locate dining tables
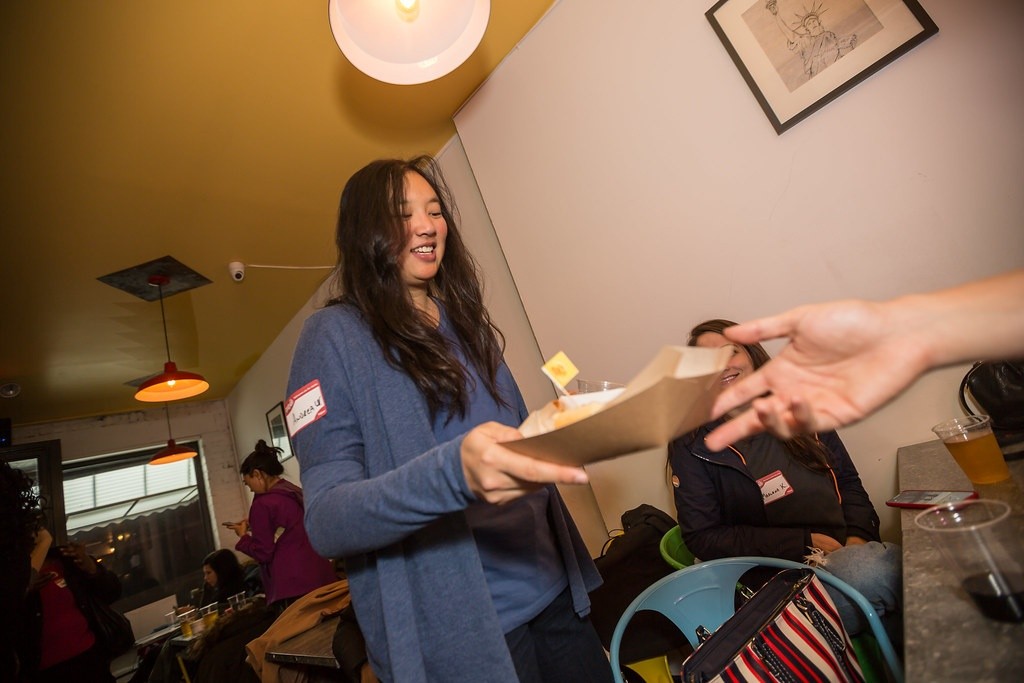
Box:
[134,613,355,683]
[896,439,1024,683]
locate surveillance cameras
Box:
[229,262,245,281]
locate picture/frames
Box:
[704,0,939,136]
[266,401,294,464]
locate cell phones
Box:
[886,488,979,511]
[222,521,238,526]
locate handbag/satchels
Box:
[959,357,1024,461]
[580,504,679,652]
[682,568,866,683]
[58,545,135,663]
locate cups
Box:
[165,610,178,629]
[176,601,219,637]
[576,378,626,393]
[227,590,247,614]
[931,414,1012,484]
[914,499,1024,623]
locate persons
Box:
[286,158,614,683]
[703,269,1024,453]
[200,549,253,614]
[0,462,121,683]
[667,319,902,630]
[226,439,330,619]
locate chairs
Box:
[127,594,180,640]
[609,525,903,683]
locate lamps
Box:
[329,0,490,86]
[134,272,209,402]
[148,402,198,464]
